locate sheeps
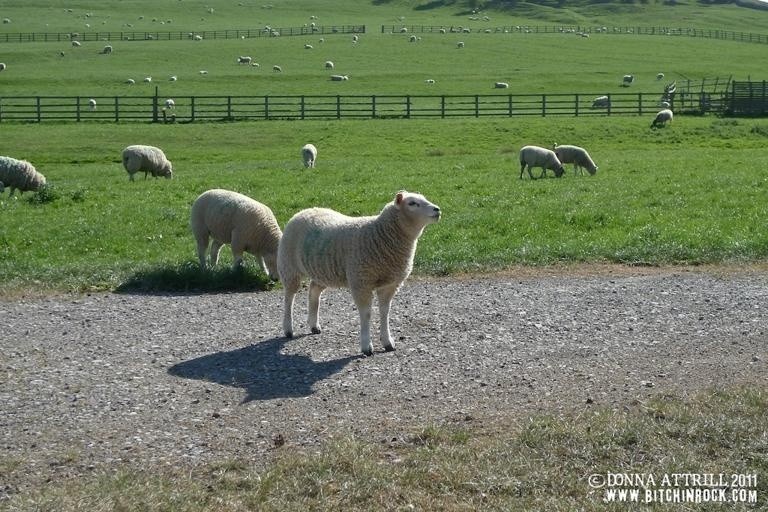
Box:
[518,142,598,180]
[650,109,674,128]
[592,95,608,108]
[301,144,317,169]
[121,144,174,182]
[191,188,284,283]
[276,189,442,356]
[494,80,508,90]
[0,156,46,197]
[657,73,664,79]
[1,25,466,110]
[622,74,634,83]
